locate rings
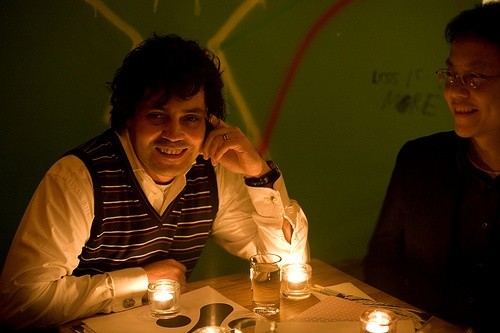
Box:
[222,133,228,139]
[214,120,226,131]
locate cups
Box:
[192,325,232,333]
[359,307,397,333]
[280,263,313,301]
[147,278,180,320]
[250,253,283,316]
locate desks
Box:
[58,259,475,333]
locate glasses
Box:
[436,68,500,89]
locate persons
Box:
[362,0,500,333]
[1,33,312,333]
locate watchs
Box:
[239,160,280,187]
